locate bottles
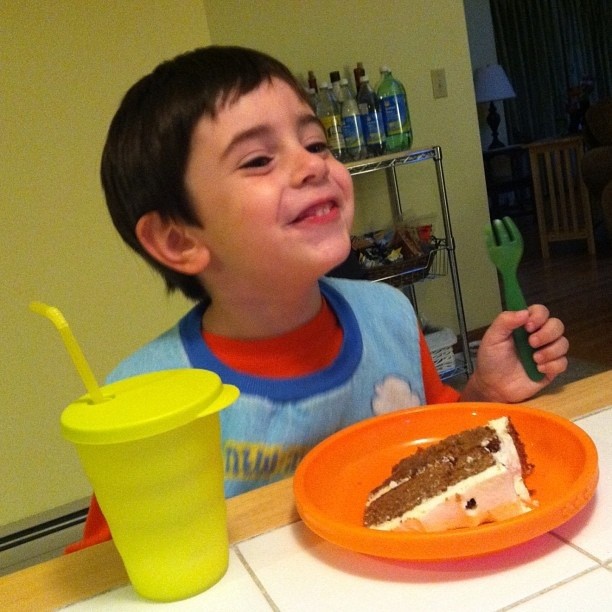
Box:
[356,75,386,158]
[307,88,318,114]
[337,79,369,161]
[305,70,320,103]
[329,71,344,113]
[354,69,378,102]
[372,66,391,93]
[317,82,346,162]
[327,83,341,124]
[377,69,413,151]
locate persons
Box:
[63,44,570,557]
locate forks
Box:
[485,217,543,382]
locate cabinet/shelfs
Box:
[343,144,473,388]
[483,143,536,220]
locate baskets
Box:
[424,325,458,376]
[344,235,437,288]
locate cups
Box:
[60,369,243,603]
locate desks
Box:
[520,132,612,262]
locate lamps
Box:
[475,61,519,147]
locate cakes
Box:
[361,414,539,533]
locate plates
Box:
[292,402,600,559]
